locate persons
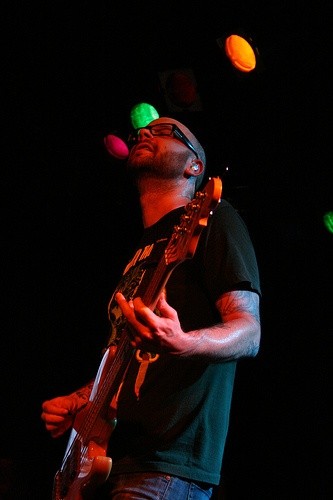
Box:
[39,110,264,499]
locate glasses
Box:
[127,123,200,161]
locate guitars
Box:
[53,177,223,500]
